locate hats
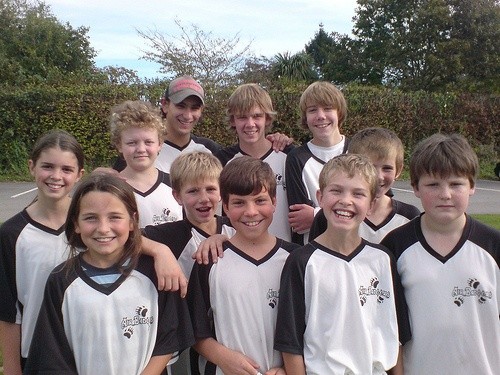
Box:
[165,74,205,107]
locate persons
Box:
[104,102,187,228]
[0,129,500,375]
[286,79,362,247]
[110,75,296,174]
[214,85,296,244]
